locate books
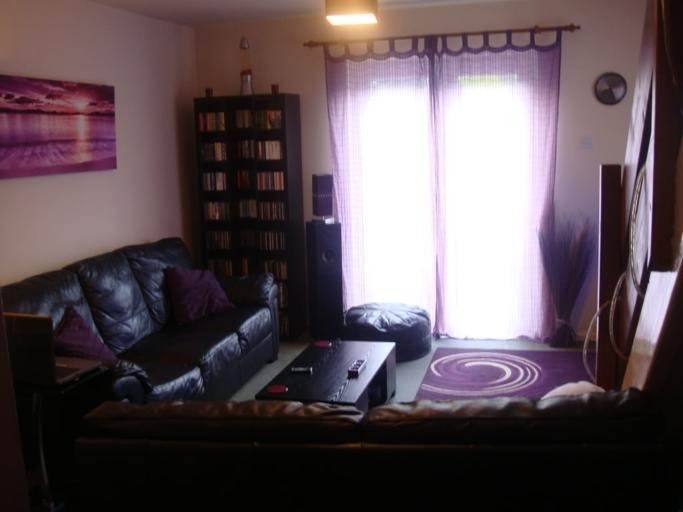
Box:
[198,109,298,338]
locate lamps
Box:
[324,1,379,27]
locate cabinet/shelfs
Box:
[193,92,307,339]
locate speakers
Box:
[306,222,344,336]
[312,174,333,216]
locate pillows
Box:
[51,304,153,394]
[164,264,236,327]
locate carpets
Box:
[412,346,596,402]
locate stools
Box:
[345,303,431,362]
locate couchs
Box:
[69,378,680,512]
[0,237,279,509]
